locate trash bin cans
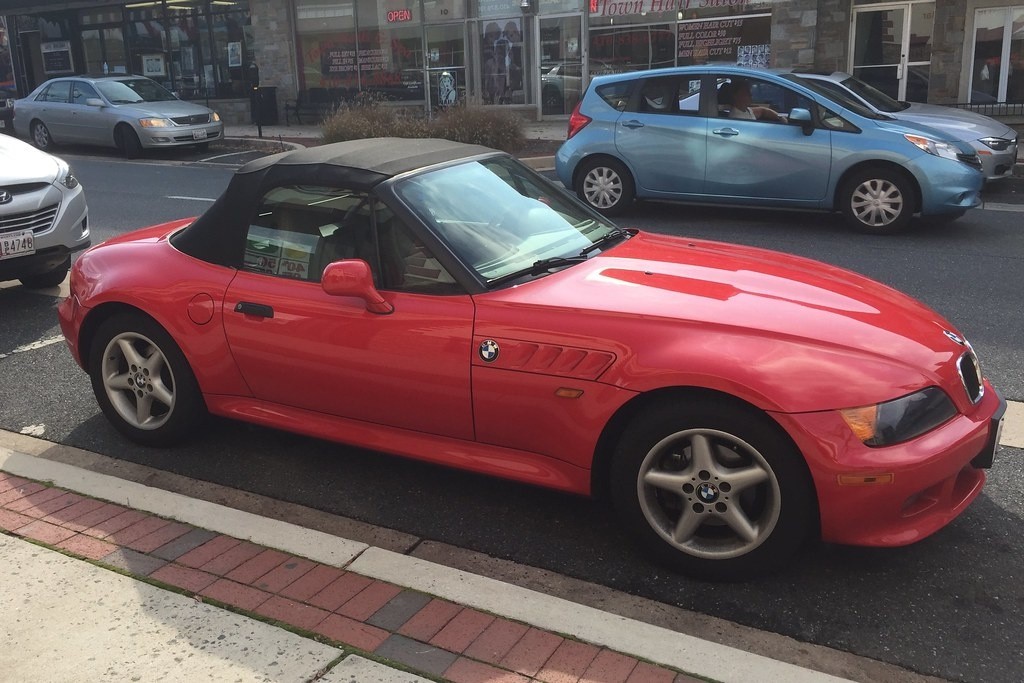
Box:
[250,86,278,126]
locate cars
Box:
[553,62,982,237]
[0,129,90,289]
[854,60,998,107]
[57,136,1008,582]
[0,89,16,131]
[9,72,227,158]
[660,69,1021,189]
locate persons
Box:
[727,75,790,126]
[480,20,527,105]
